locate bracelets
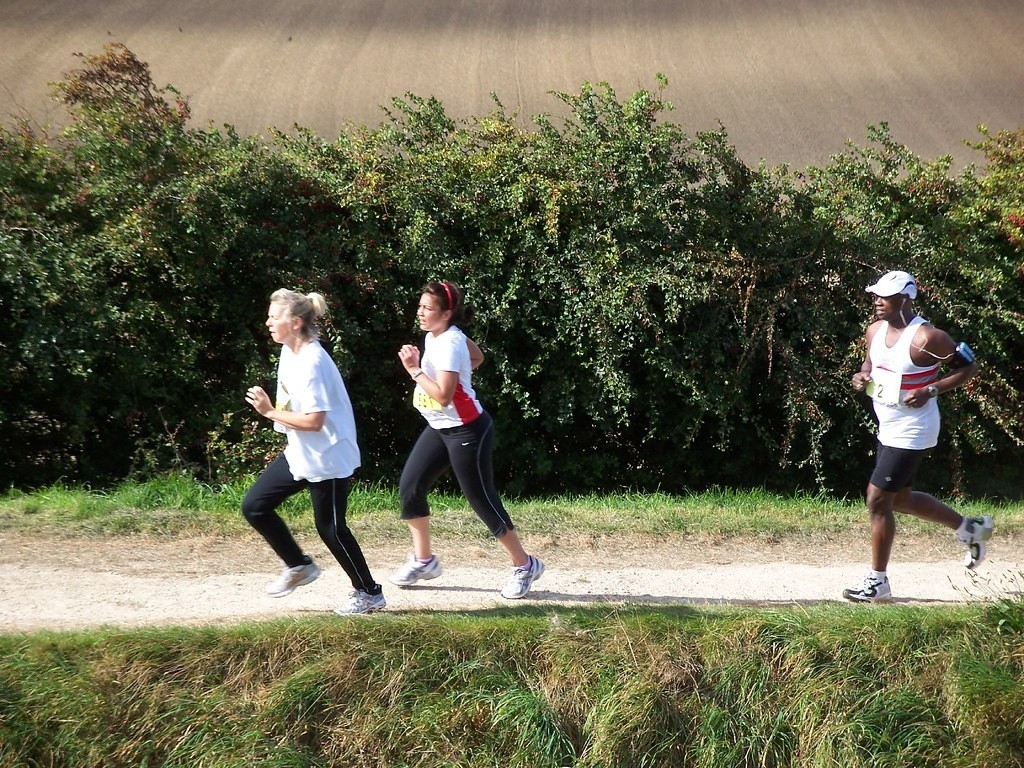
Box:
[411,369,423,379]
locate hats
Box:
[865,270,918,300]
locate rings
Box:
[856,384,860,388]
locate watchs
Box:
[927,385,938,398]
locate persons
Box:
[842,271,992,603]
[389,282,545,599]
[240,288,387,617]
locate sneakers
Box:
[964,515,992,570]
[333,590,387,616]
[843,575,891,603]
[390,553,443,586]
[265,562,321,599]
[500,555,546,599]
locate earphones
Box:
[902,298,907,302]
